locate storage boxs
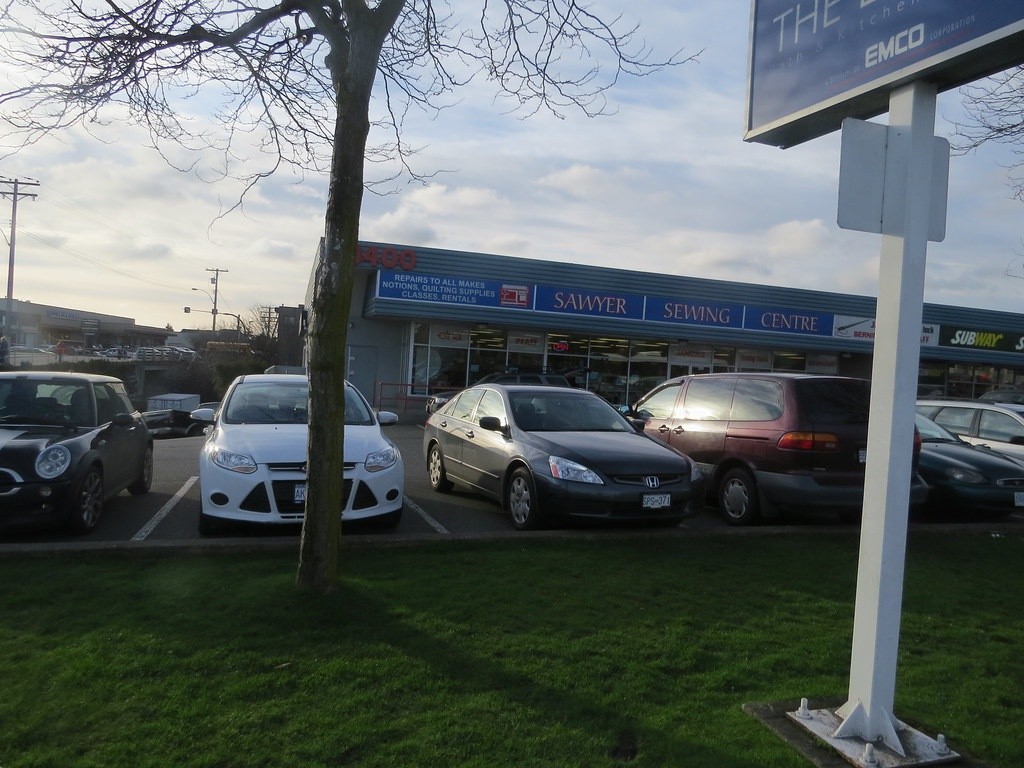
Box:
[147,393,200,412]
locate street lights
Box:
[192,288,218,332]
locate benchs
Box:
[269,400,307,420]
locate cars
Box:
[422,382,708,533]
[910,409,1024,521]
[187,372,405,537]
[0,370,155,535]
[10,340,196,362]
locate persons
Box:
[117,347,128,360]
[68,388,99,426]
[0,380,49,423]
[57,339,64,363]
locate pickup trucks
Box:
[139,401,221,440]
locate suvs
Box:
[610,372,922,527]
[979,388,1024,406]
[424,366,578,418]
[916,395,1024,461]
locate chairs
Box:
[66,389,107,427]
[237,393,275,421]
[516,403,543,431]
[0,385,46,419]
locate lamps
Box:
[348,321,353,328]
